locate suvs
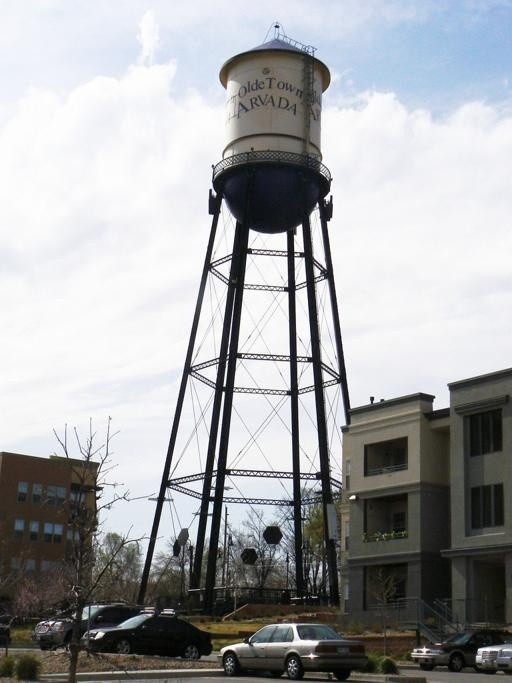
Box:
[34,602,142,650]
[476,634,512,675]
[410,630,512,673]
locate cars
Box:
[81,613,213,660]
[216,621,369,680]
